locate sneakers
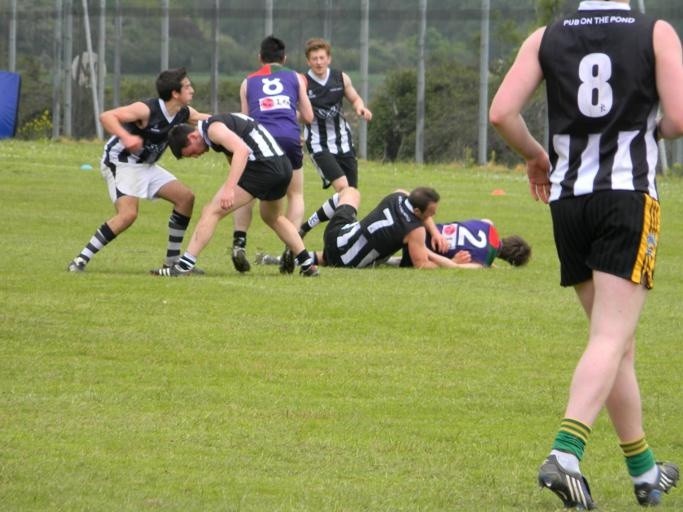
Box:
[538,454,593,511]
[151,261,204,276]
[634,461,679,507]
[68,261,83,273]
[231,246,319,276]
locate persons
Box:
[66,66,212,276]
[384,217,531,270]
[299,38,372,193]
[254,185,471,270]
[149,111,320,277]
[490,0,683,512]
[240,35,315,275]
